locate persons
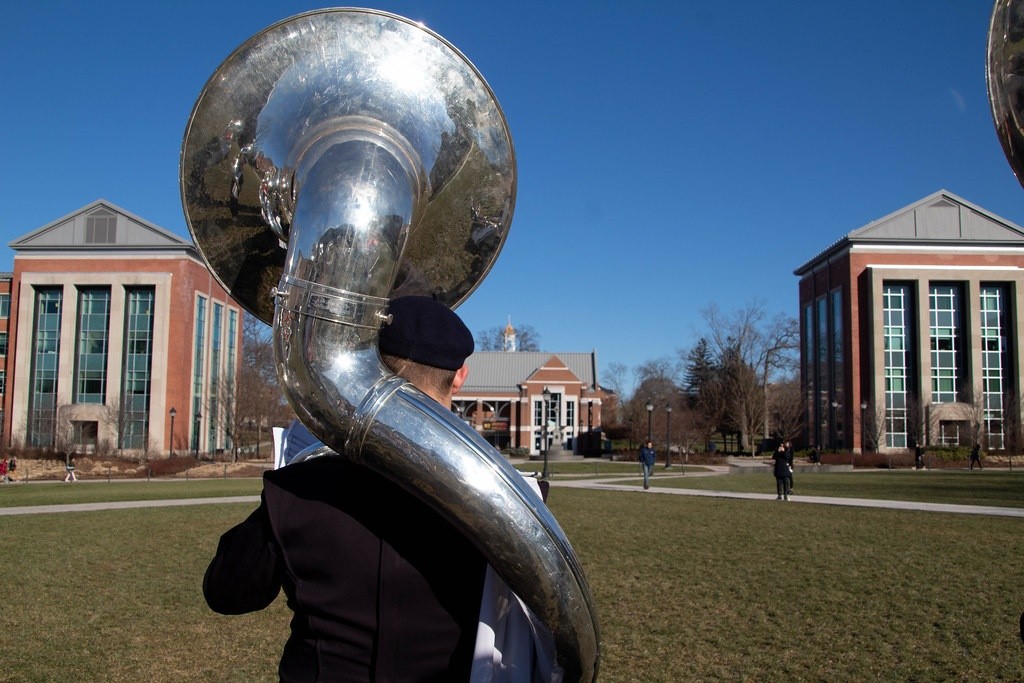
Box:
[65,457,78,482]
[0,458,8,482]
[784,440,794,494]
[202,295,558,683]
[970,444,982,470]
[9,456,19,481]
[810,447,821,466]
[640,441,656,489]
[772,438,791,500]
[912,441,926,470]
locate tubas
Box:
[176,5,603,682]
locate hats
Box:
[378,293,474,371]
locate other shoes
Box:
[644,485,648,489]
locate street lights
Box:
[665,402,673,469]
[195,412,202,461]
[170,407,176,458]
[542,388,552,480]
[645,398,654,442]
[861,401,867,454]
[832,398,838,452]
